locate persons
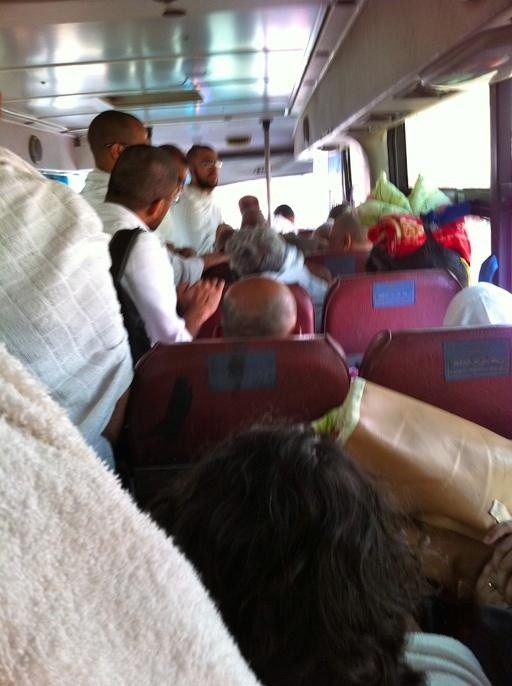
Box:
[2,146,134,475]
[163,421,489,683]
[213,277,303,338]
[2,341,262,683]
[78,111,151,207]
[225,224,332,334]
[153,145,234,258]
[238,196,374,257]
[359,170,471,288]
[482,520,510,606]
[444,281,510,328]
[96,145,230,367]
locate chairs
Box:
[359,323,511,435]
[124,336,351,464]
[325,269,464,357]
[117,228,377,338]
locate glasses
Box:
[200,160,225,170]
